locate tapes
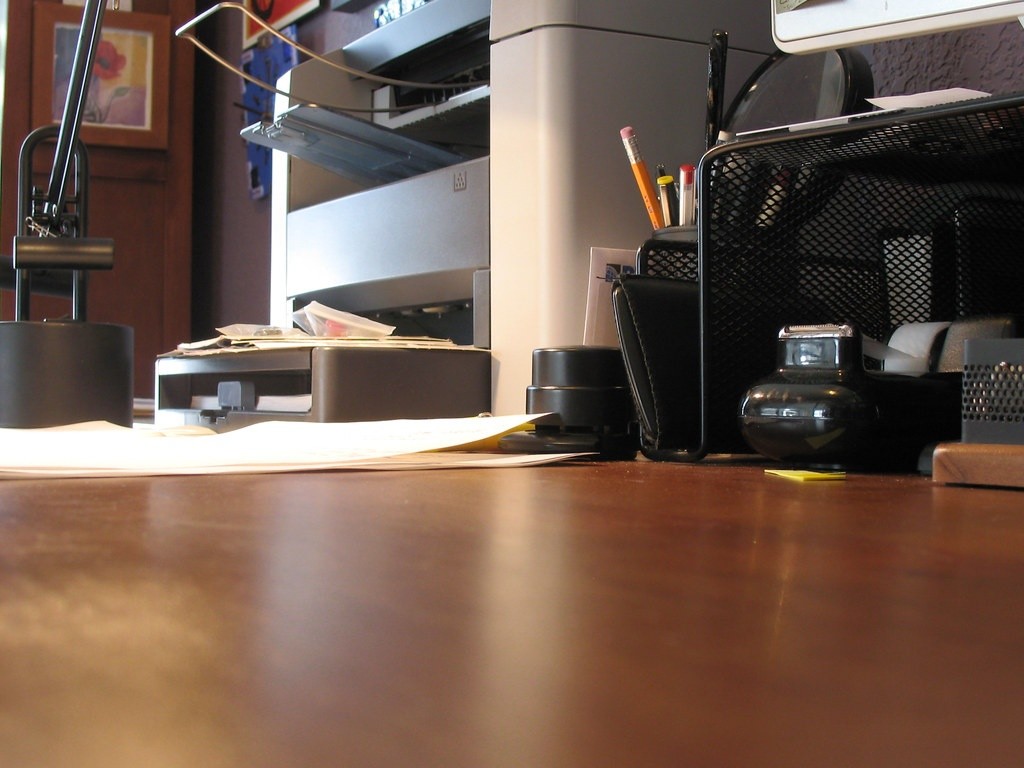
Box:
[882,321,952,376]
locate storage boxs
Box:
[962,337,1024,445]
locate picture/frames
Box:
[241,0,324,51]
[30,2,172,151]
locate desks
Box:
[0,409,1024,768]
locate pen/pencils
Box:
[620,29,736,231]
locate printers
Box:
[154,0,826,440]
